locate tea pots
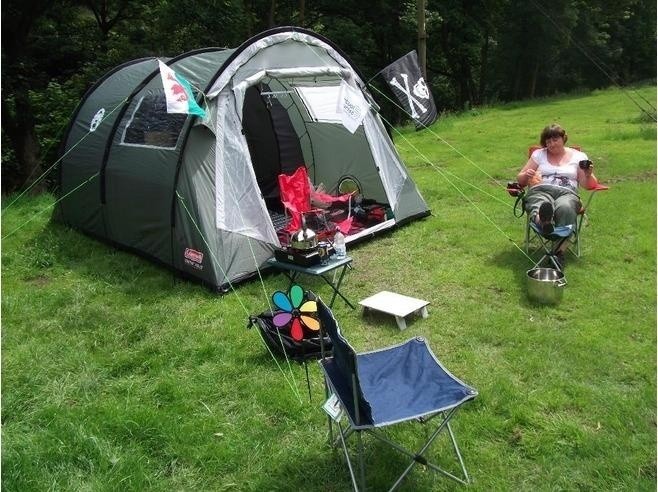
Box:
[290,215,318,252]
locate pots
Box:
[526,267,567,304]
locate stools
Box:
[358,289,432,331]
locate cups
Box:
[579,160,590,169]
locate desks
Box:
[267,254,355,313]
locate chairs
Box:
[507,145,609,278]
[313,295,482,490]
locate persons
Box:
[517,125,598,258]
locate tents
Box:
[51,26,431,293]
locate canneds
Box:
[318,243,328,266]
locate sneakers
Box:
[539,200,555,234]
[551,250,566,269]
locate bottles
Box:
[332,225,347,260]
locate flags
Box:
[335,79,372,135]
[381,50,440,131]
[158,59,207,118]
